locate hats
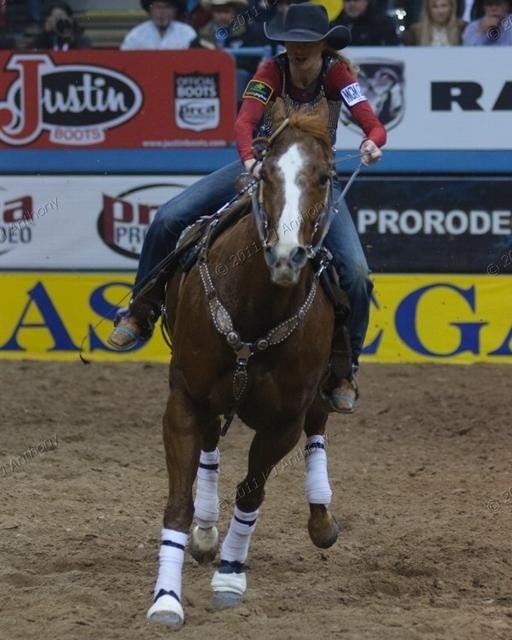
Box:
[200,0,248,13]
[262,4,351,51]
[140,0,187,16]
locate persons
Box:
[119,1,511,50]
[106,1,389,416]
[0,1,90,49]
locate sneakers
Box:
[328,377,359,415]
[107,311,152,351]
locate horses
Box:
[145,96,339,630]
